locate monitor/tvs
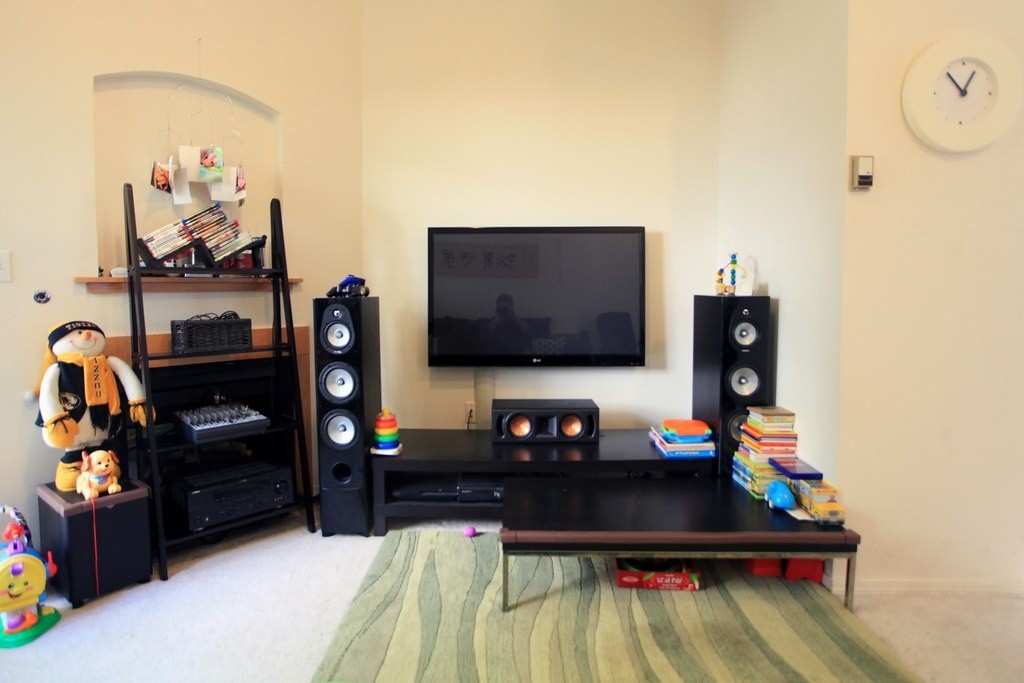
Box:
[427,228,646,367]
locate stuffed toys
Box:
[25,321,156,502]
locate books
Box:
[142,204,252,262]
[732,406,797,500]
[648,426,716,459]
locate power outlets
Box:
[463,401,477,423]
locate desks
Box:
[498,476,862,614]
[370,428,716,535]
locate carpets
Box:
[310,529,926,683]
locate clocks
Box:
[900,30,1024,155]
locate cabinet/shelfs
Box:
[122,183,317,582]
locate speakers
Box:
[491,399,600,445]
[692,295,773,477]
[313,296,382,533]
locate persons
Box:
[235,175,246,193]
[200,148,223,179]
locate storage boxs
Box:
[614,558,701,591]
